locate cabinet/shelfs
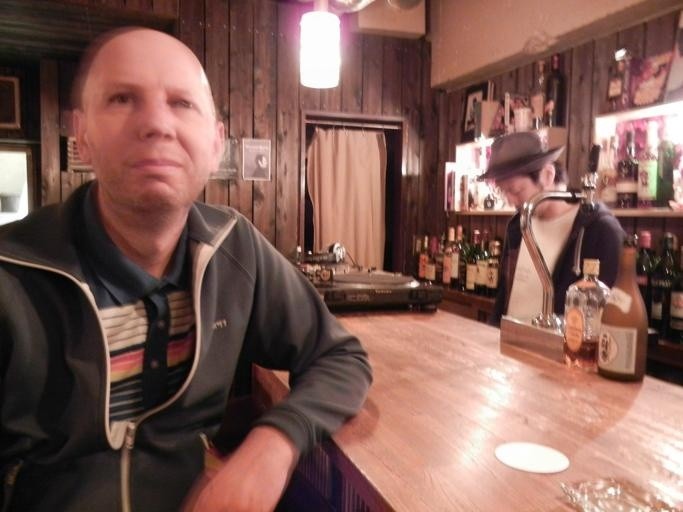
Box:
[448,125,567,217]
[435,286,682,387]
[585,96,681,219]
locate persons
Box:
[476,131,628,328]
[252,155,267,178]
[0,26,375,512]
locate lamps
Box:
[297,0,341,90]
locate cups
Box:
[512,107,532,132]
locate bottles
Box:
[594,234,647,384]
[562,259,610,369]
[580,130,682,208]
[636,230,682,347]
[531,53,567,127]
[413,224,501,296]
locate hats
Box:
[477,132,566,181]
